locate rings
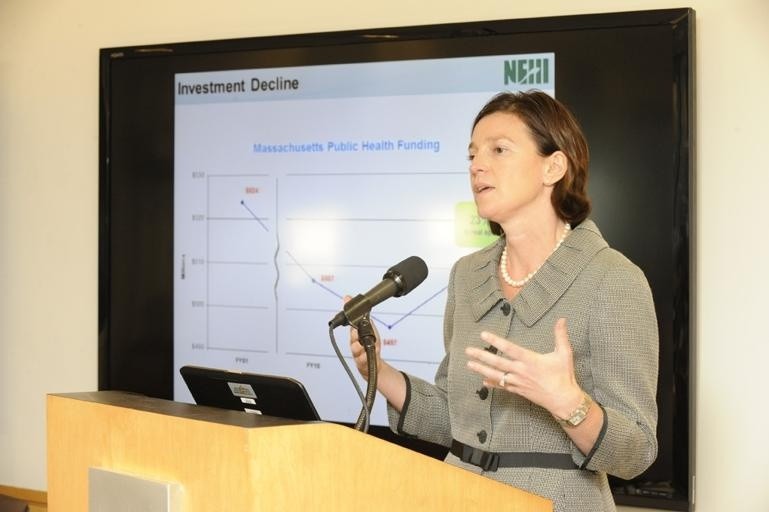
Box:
[498,369,513,390]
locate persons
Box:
[343,87,659,512]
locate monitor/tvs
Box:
[98,6,695,512]
[179,365,321,421]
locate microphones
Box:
[328,256,429,330]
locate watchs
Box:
[552,391,594,430]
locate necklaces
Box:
[499,219,573,288]
